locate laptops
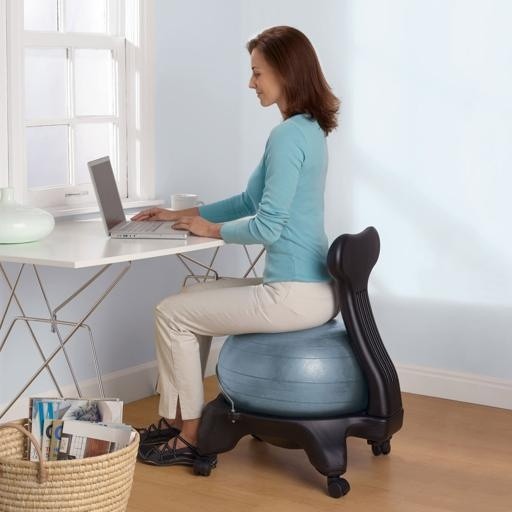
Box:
[87,155,191,240]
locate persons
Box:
[130,26,342,468]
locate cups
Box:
[171,194,204,210]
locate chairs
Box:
[192,227,405,500]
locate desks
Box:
[1,208,266,423]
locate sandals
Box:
[132,415,182,444]
[138,433,218,468]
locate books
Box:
[26,398,134,465]
